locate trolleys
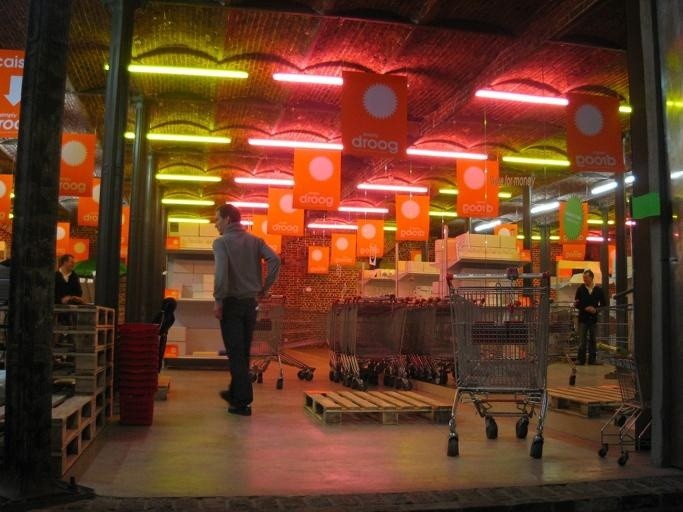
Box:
[326,295,488,392]
[248,294,316,390]
[592,302,652,466]
[517,300,579,386]
[445,266,551,459]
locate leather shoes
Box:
[574,359,585,365]
[220,390,239,406]
[227,406,253,416]
[588,360,600,365]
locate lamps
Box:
[586,169,638,200]
[404,145,490,162]
[127,62,252,81]
[148,166,516,241]
[122,128,234,147]
[518,191,565,219]
[472,85,570,107]
[501,153,571,170]
[269,67,412,92]
[616,104,635,116]
[245,132,343,153]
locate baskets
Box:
[115,322,160,427]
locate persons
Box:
[572,269,608,366]
[210,202,281,417]
[54,253,88,365]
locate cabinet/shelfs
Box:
[51,306,115,477]
